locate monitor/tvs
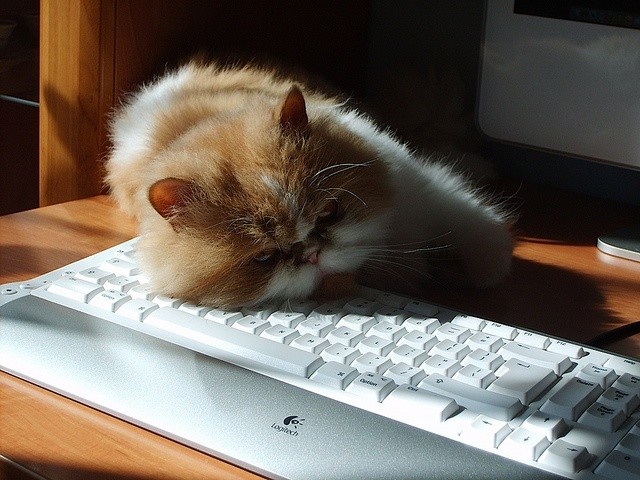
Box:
[474,1,639,261]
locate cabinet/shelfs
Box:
[1,1,210,210]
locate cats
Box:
[102,52,526,312]
